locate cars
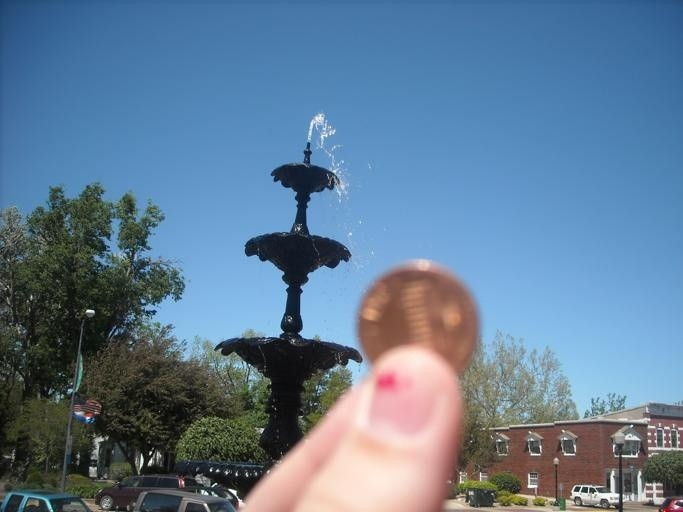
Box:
[657,496,683,512]
[570,485,626,509]
[0,475,239,512]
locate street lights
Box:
[60,308,95,493]
[614,430,625,512]
[553,456,560,505]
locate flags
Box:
[73,398,101,425]
[75,353,82,392]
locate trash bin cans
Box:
[468,488,496,507]
[560,497,566,510]
[89,460,98,480]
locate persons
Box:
[236,345,464,511]
